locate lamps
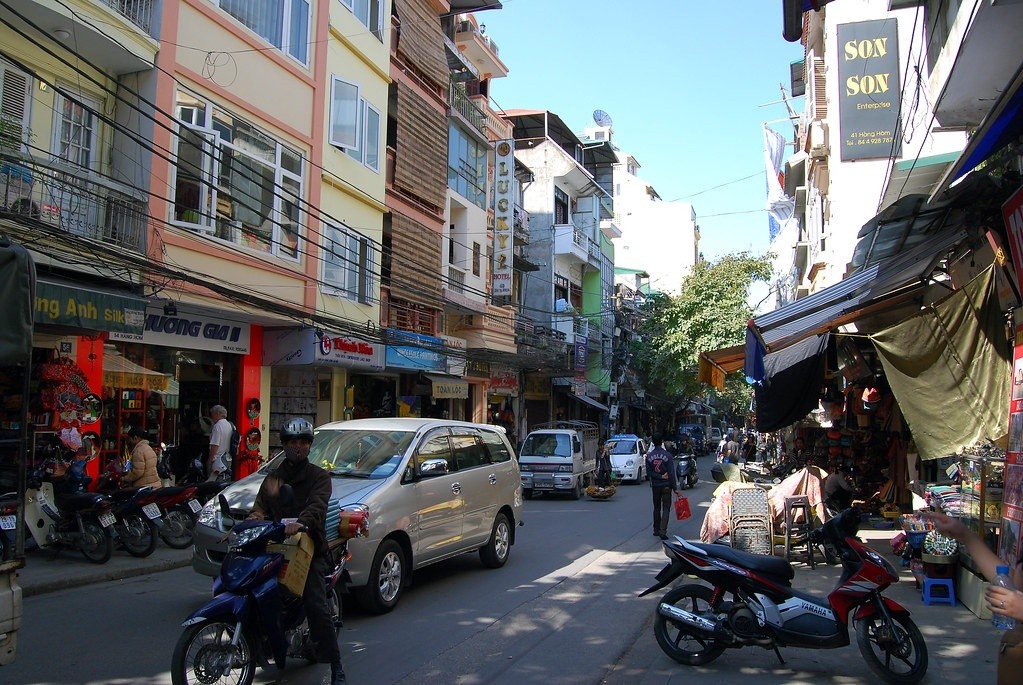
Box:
[163,298,178,315]
[479,22,486,35]
[51,27,73,41]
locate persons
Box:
[824,465,854,508]
[789,437,816,471]
[119,427,162,492]
[922,511,1023,685]
[221,418,347,685]
[675,438,696,478]
[646,432,682,540]
[715,433,769,465]
[596,437,612,500]
[207,405,242,482]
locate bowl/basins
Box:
[921,552,957,578]
[905,530,928,547]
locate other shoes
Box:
[659,533,669,540]
[653,532,659,536]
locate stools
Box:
[783,495,817,570]
[921,576,958,608]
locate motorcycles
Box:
[170,492,352,685]
[0,441,236,565]
[638,491,929,685]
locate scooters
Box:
[673,453,700,490]
[710,452,862,565]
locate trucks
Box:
[675,414,713,457]
[517,419,600,500]
[710,419,726,452]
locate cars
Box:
[606,439,649,485]
[191,417,525,614]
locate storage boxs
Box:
[266,531,315,598]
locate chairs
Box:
[728,487,775,556]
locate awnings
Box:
[34,275,149,335]
[423,375,469,399]
[699,222,968,392]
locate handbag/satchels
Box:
[231,423,238,454]
[674,493,691,520]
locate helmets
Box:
[279,418,315,439]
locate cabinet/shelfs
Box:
[960,453,1005,575]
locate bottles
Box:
[992,565,1016,631]
